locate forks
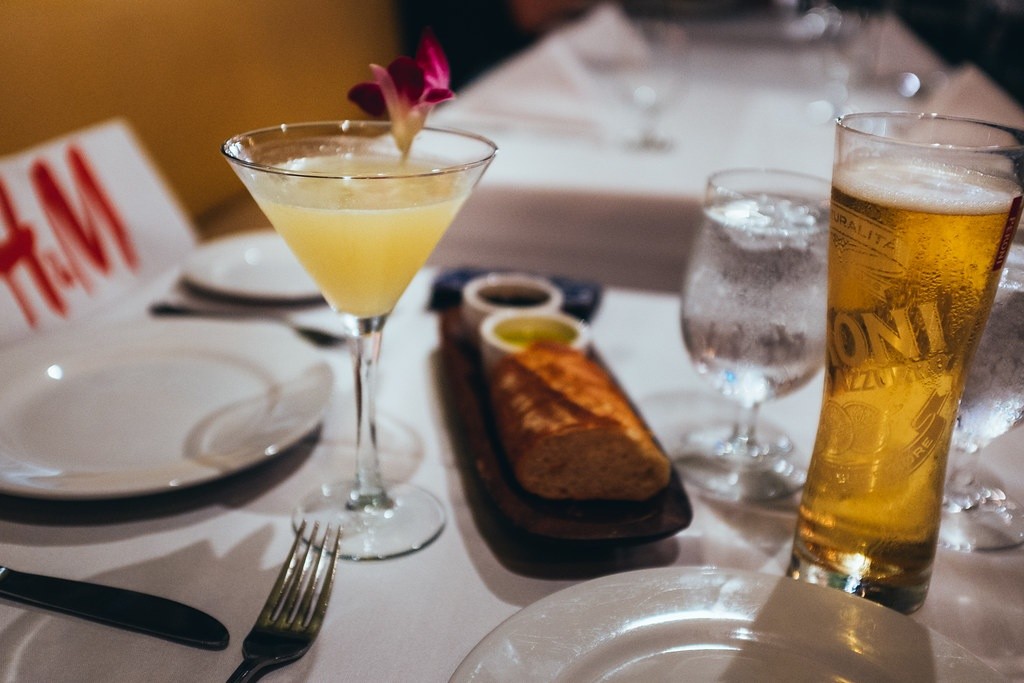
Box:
[225,519,343,683]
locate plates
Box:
[448,566,1014,683]
[182,230,322,301]
[443,289,693,544]
[0,317,336,498]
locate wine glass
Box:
[928,237,1024,552]
[219,121,500,560]
[679,169,838,501]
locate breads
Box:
[485,338,671,502]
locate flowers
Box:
[345,37,457,158]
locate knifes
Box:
[1,566,229,652]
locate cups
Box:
[786,112,1024,616]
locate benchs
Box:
[0,0,398,242]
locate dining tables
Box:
[0,5,1024,683]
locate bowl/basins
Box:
[480,311,592,368]
[463,271,564,333]
